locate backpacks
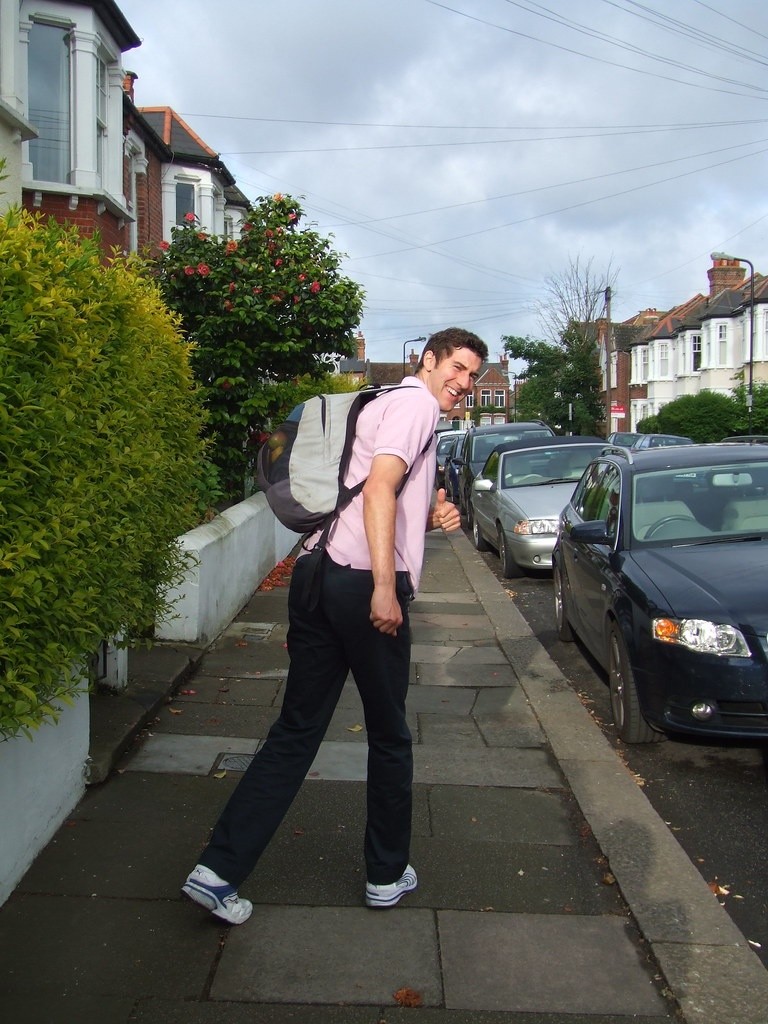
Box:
[256,384,433,532]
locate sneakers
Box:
[366,864,417,908]
[181,864,253,925]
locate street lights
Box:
[402,336,427,379]
[710,251,754,435]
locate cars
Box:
[435,429,465,506]
[469,436,616,579]
[717,436,768,447]
[606,431,645,453]
[629,434,705,494]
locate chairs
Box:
[634,500,696,539]
[563,451,592,478]
[721,495,768,532]
[510,458,532,474]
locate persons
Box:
[178,328,488,925]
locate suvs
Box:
[548,435,768,745]
[450,420,556,533]
